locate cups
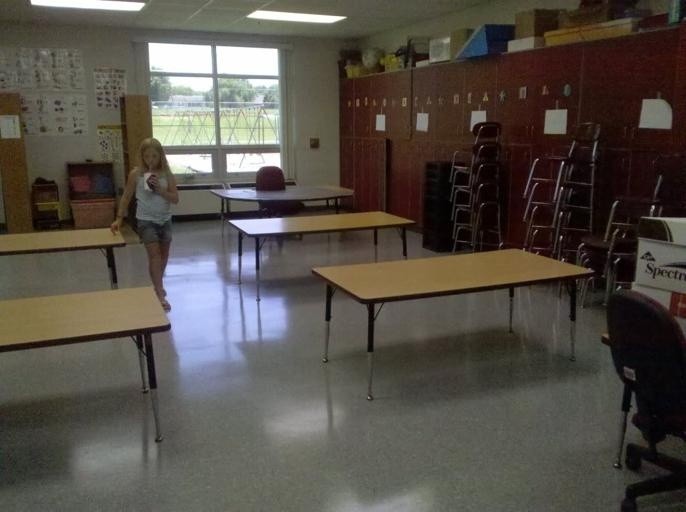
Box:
[144,173,158,191]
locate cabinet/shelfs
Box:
[1,92,33,235]
[339,69,413,225]
[118,94,153,231]
[414,25,686,286]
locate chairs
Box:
[602,286,686,511]
[257,166,302,240]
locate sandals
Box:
[159,294,171,311]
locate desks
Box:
[310,248,593,400]
[71,200,116,231]
[211,182,357,250]
[0,285,170,446]
[227,212,417,302]
[0,226,128,287]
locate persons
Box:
[109,137,179,312]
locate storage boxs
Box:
[427,2,670,62]
[30,183,59,232]
[631,214,686,338]
